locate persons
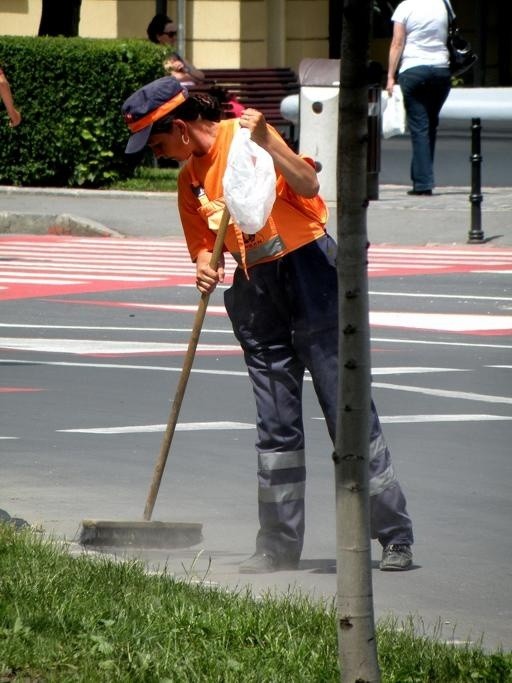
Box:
[0,68,22,128]
[384,0,456,196]
[122,74,415,577]
[146,12,207,169]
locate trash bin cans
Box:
[299,56,385,202]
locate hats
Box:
[124,77,189,155]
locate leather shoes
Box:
[407,188,432,196]
[379,544,415,570]
[238,553,300,575]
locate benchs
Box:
[152,67,302,169]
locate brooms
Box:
[81,204,229,551]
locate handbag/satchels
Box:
[447,17,480,78]
[379,84,407,139]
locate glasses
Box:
[162,30,178,39]
